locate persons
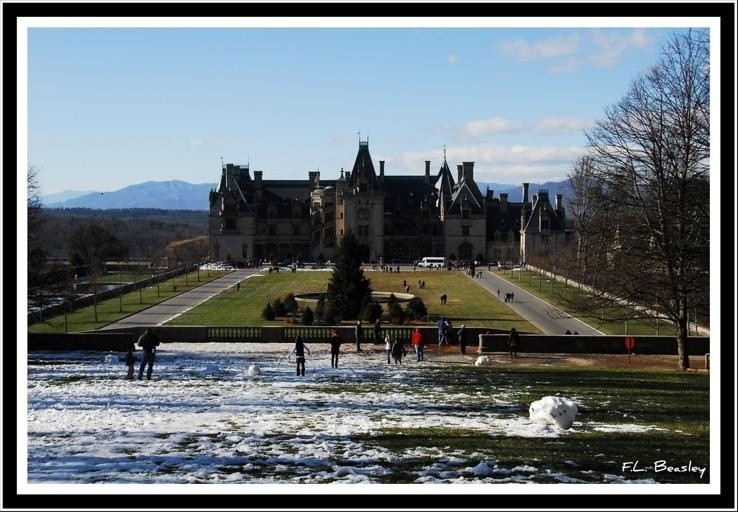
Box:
[355,315,468,365]
[565,329,571,335]
[403,280,425,293]
[124,346,137,378]
[329,331,341,368]
[136,331,159,380]
[441,295,447,304]
[413,252,517,279]
[497,288,514,303]
[506,327,520,358]
[574,331,579,335]
[370,263,400,274]
[288,335,310,376]
[241,256,301,274]
[237,283,240,291]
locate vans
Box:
[498,262,511,269]
[418,256,446,268]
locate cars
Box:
[512,265,527,272]
[218,264,234,270]
[488,260,497,266]
